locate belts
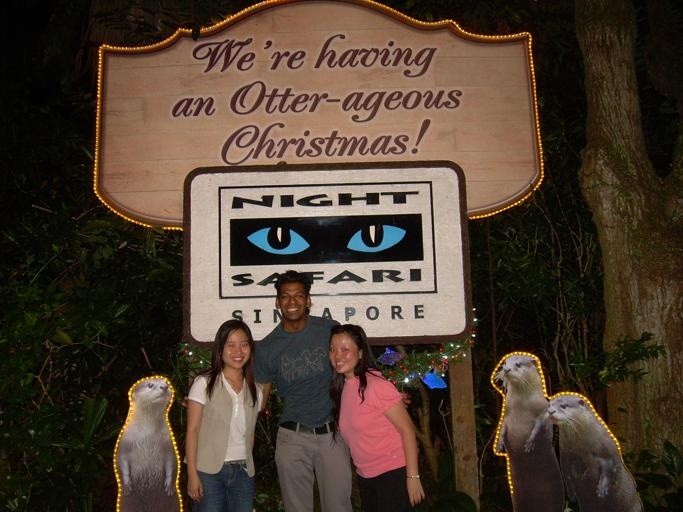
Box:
[279,420,336,435]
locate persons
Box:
[327,323,427,512]
[180,269,412,510]
[179,319,264,512]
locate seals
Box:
[116,377,180,512]
[545,393,644,511]
[492,354,568,512]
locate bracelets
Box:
[405,475,419,479]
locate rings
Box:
[192,494,196,497]
